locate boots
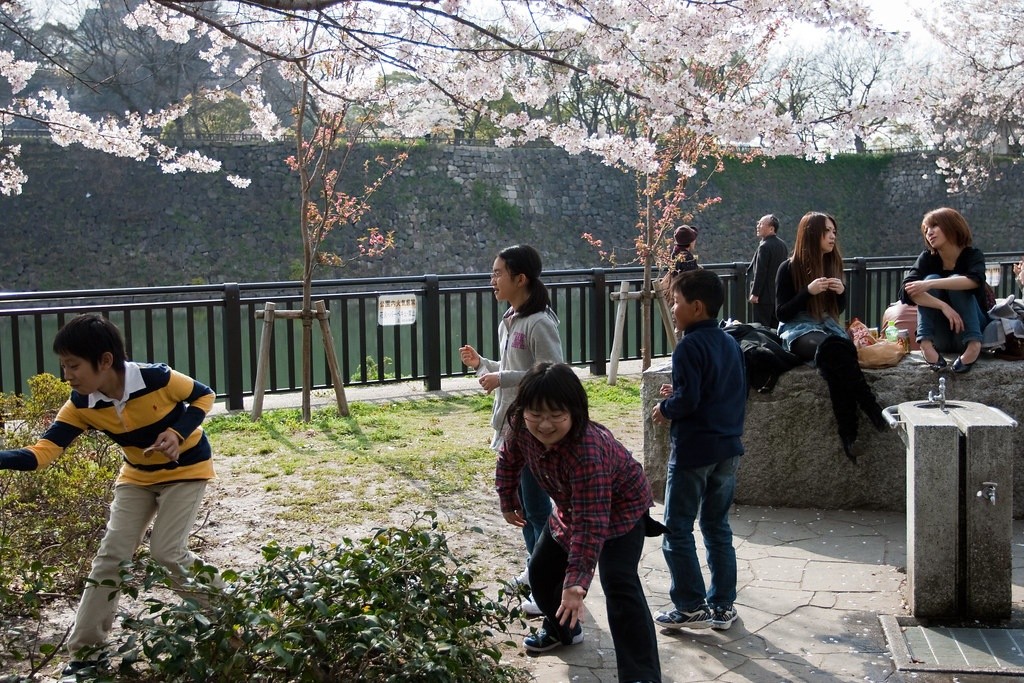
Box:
[814,335,891,458]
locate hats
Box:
[673,225,700,245]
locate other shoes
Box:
[921,346,948,373]
[518,593,545,615]
[950,354,979,374]
[503,567,531,594]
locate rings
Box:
[835,284,839,288]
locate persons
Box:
[900,206,990,373]
[647,269,751,634]
[774,208,896,462]
[494,358,676,683]
[748,212,787,334]
[1011,253,1024,301]
[663,224,708,341]
[453,243,584,656]
[2,312,241,683]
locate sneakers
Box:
[711,606,737,630]
[653,604,713,630]
[522,621,583,651]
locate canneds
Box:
[897,329,912,354]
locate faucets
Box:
[928,376,949,413]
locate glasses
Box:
[522,409,571,424]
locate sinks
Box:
[912,399,970,415]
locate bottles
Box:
[885,321,898,344]
